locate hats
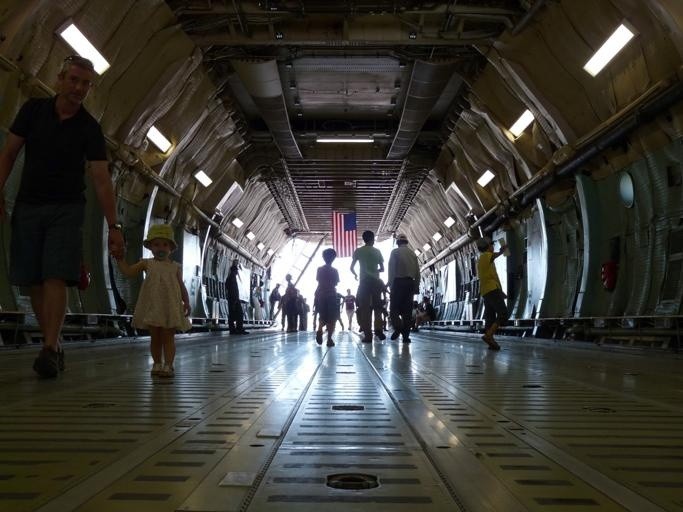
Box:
[396,234,409,243]
[476,237,497,247]
[142,224,179,251]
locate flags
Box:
[331,210,358,258]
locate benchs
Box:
[1,285,138,350]
[473,292,683,357]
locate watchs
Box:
[107,221,123,230]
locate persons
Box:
[108,223,193,379]
[384,233,421,344]
[224,265,250,336]
[313,247,340,346]
[475,236,510,351]
[349,231,386,343]
[0,52,129,381]
[249,274,436,333]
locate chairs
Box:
[418,300,470,331]
[190,296,275,331]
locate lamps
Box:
[582,18,639,79]
[54,18,112,78]
[192,168,212,188]
[244,230,255,241]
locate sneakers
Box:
[162,366,174,377]
[403,337,412,344]
[230,329,249,335]
[33,352,61,379]
[481,335,500,350]
[361,335,372,343]
[327,341,335,347]
[390,331,399,340]
[286,329,306,333]
[376,334,386,341]
[151,363,161,373]
[316,331,322,344]
